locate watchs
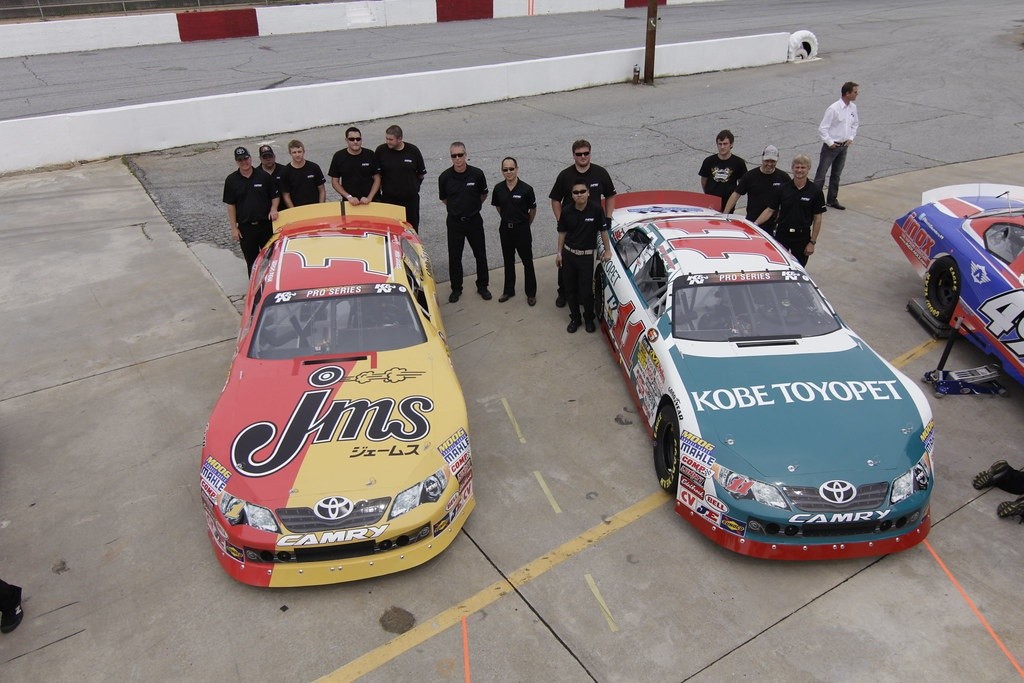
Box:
[809,241,816,245]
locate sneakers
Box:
[973,460,1009,490]
[997,496,1024,518]
[556,296,567,308]
[448,291,462,303]
[477,288,492,300]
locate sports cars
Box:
[200,201,478,588]
[594,190,934,561]
[891,182,1024,392]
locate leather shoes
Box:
[499,294,515,302]
[528,297,536,306]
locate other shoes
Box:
[567,313,582,333]
[583,311,597,333]
[826,196,846,210]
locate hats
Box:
[234,146,248,160]
[259,145,274,157]
[762,145,779,161]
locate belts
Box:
[238,219,269,225]
[564,244,593,256]
[500,221,527,228]
[834,142,846,147]
[778,224,810,233]
[448,214,480,221]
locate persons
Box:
[549,140,616,307]
[438,142,493,303]
[255,144,286,212]
[555,181,611,333]
[753,156,826,268]
[327,127,382,206]
[699,130,748,214]
[222,147,281,279]
[0,579,23,633]
[813,82,859,210]
[492,157,537,307]
[723,145,791,236]
[375,125,427,235]
[280,139,326,209]
[973,460,1024,525]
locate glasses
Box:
[261,154,274,159]
[572,189,588,194]
[237,156,249,161]
[451,153,465,158]
[575,151,591,156]
[347,137,362,142]
[503,167,516,172]
